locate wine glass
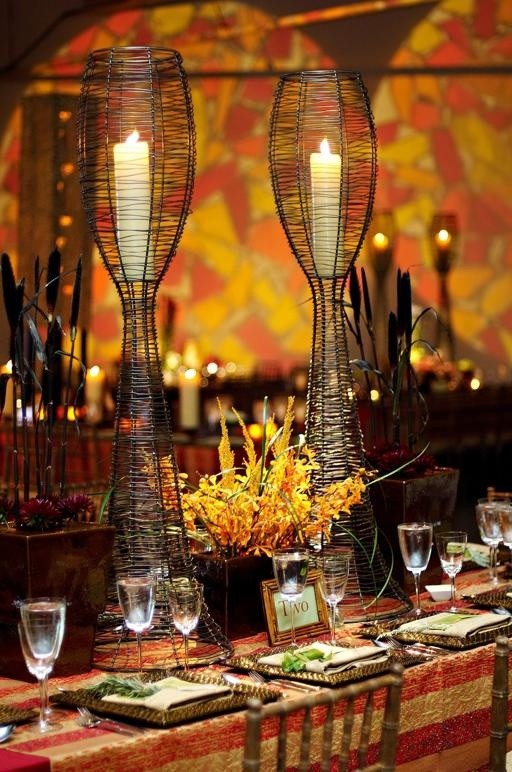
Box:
[434,531,468,614]
[270,547,310,646]
[168,581,205,673]
[17,595,69,733]
[475,496,512,588]
[117,577,158,674]
[316,555,351,645]
[397,522,435,616]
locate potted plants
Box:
[1,248,114,678]
[324,258,460,600]
[137,397,376,646]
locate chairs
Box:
[242,664,408,772]
[487,488,511,508]
[487,633,512,772]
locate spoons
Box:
[370,639,430,656]
[0,724,17,742]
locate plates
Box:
[423,583,458,602]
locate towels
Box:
[101,675,233,715]
[257,639,389,675]
[396,611,511,637]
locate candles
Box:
[113,129,155,281]
[306,137,345,276]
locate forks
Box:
[247,670,317,696]
[385,634,450,656]
[78,705,150,737]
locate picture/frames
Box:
[260,569,331,647]
